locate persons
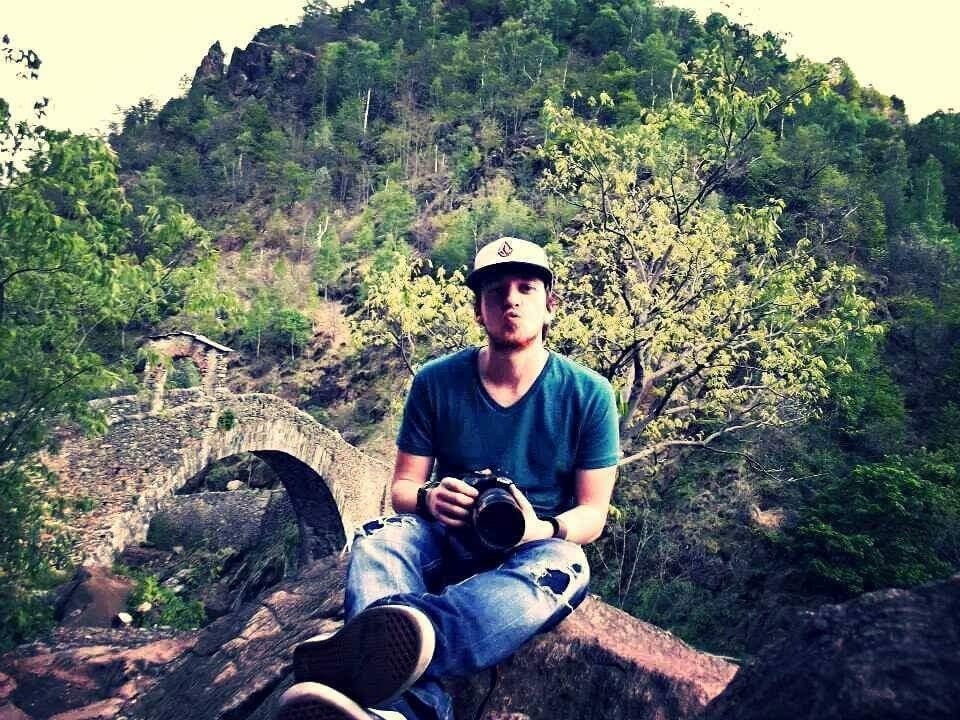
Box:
[278,237,620,720]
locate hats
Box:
[464,237,557,285]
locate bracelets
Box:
[540,516,560,537]
[415,480,440,523]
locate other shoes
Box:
[272,682,407,720]
[291,603,435,708]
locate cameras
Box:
[460,469,526,553]
